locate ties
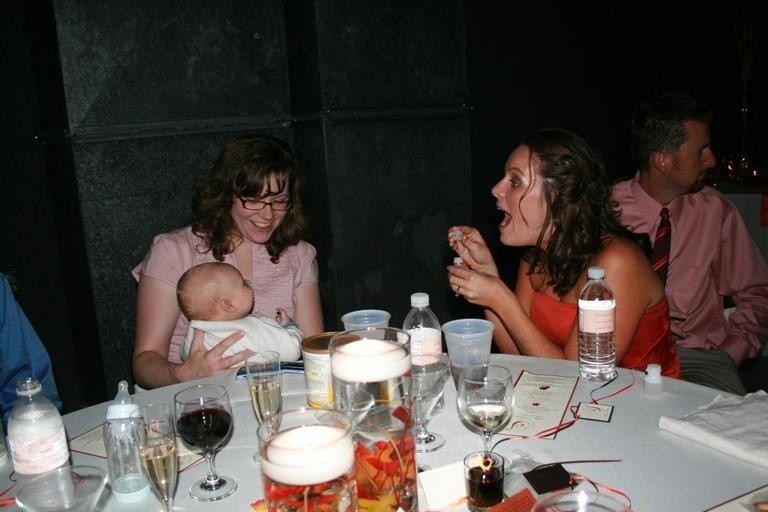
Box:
[650,208,671,288]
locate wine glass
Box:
[131,399,184,512]
[244,348,282,463]
[456,364,516,449]
[171,380,239,502]
[398,349,445,452]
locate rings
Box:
[455,286,460,297]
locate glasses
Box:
[236,195,293,211]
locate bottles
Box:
[576,267,617,381]
[453,257,469,297]
[642,363,665,397]
[101,380,151,503]
[7,376,76,508]
[400,291,442,371]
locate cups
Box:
[463,450,504,512]
[327,326,419,512]
[256,407,358,511]
[16,462,107,511]
[529,488,629,511]
[439,318,496,390]
[338,308,393,339]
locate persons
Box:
[446,126,680,378]
[176,263,305,374]
[606,90,768,396]
[0,270,63,430]
[129,134,326,389]
